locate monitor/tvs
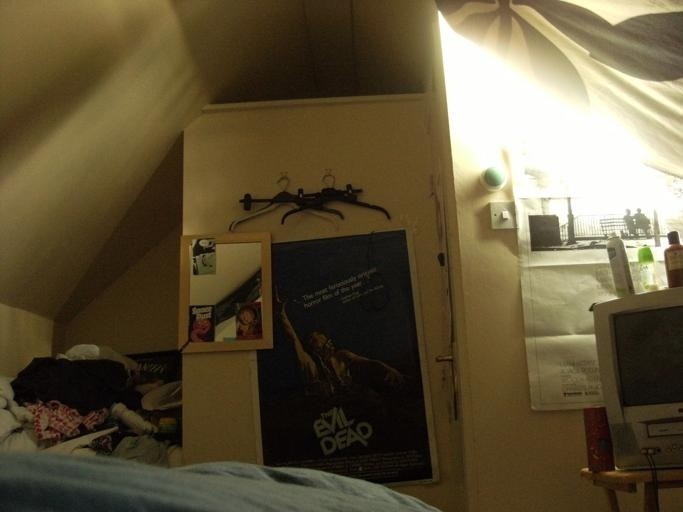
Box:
[592,287,683,471]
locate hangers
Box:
[230,174,391,232]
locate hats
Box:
[141,379,182,411]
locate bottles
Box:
[663,231,683,288]
[637,246,660,293]
[606,231,636,299]
[583,405,615,473]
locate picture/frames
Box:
[178,232,274,354]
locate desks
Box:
[580,469,682,511]
